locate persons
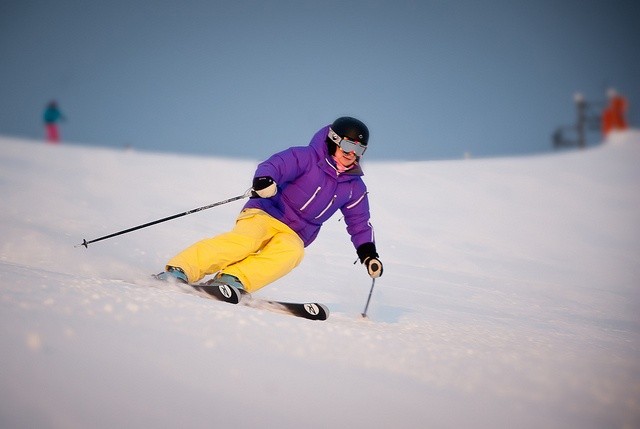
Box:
[599,86,626,133]
[76,116,383,299]
[41,100,67,144]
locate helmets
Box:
[332,117,369,145]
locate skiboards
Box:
[151,272,330,321]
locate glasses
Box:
[328,127,367,157]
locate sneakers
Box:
[158,267,188,283]
[206,273,246,304]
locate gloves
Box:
[364,255,383,278]
[253,176,277,198]
